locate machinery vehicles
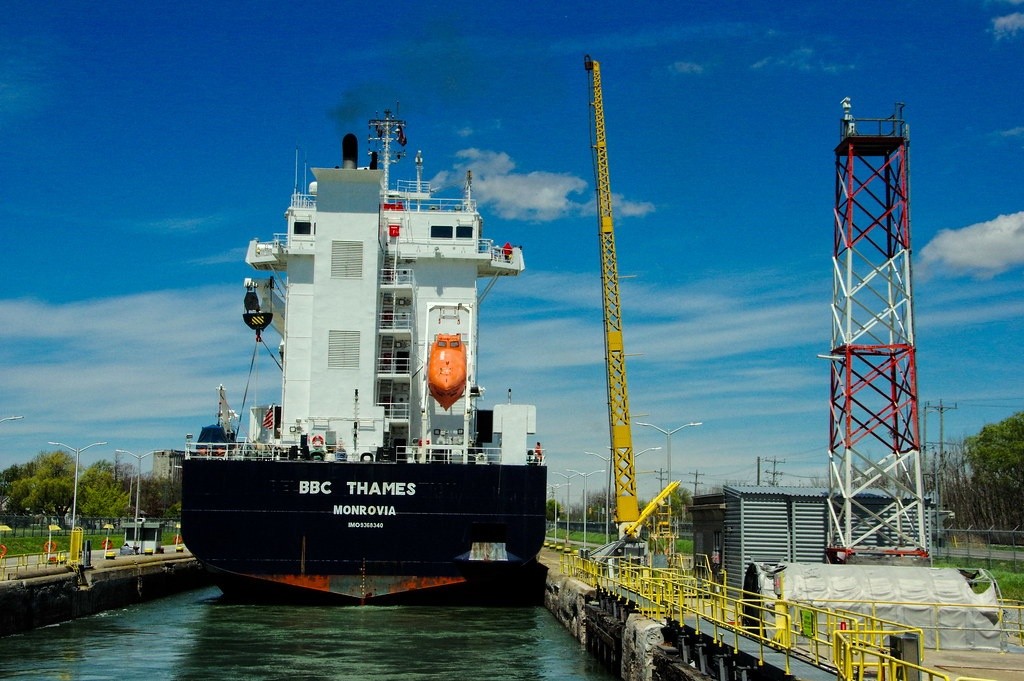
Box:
[582,51,681,581]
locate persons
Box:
[536,441,543,466]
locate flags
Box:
[262,406,274,430]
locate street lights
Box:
[603,445,661,475]
[565,469,605,548]
[546,483,573,544]
[585,450,627,546]
[635,422,703,520]
[48,441,109,531]
[552,472,585,543]
[116,449,168,547]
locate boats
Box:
[429,332,467,410]
[181,99,549,605]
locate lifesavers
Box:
[43,540,56,554]
[0,545,7,560]
[173,534,184,545]
[311,435,325,452]
[102,538,112,549]
